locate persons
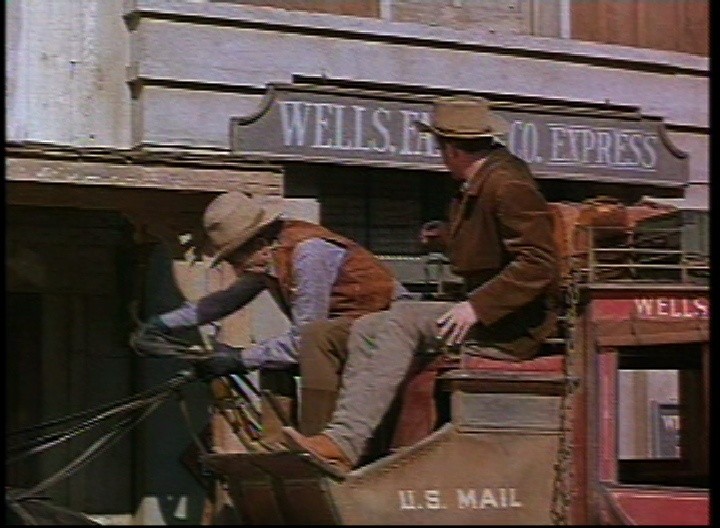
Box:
[128,191,410,453]
[283,94,566,484]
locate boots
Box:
[245,393,299,453]
[277,382,335,451]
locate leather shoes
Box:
[279,425,352,480]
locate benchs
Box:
[407,331,566,382]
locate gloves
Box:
[189,348,249,383]
[129,312,174,356]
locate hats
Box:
[413,94,507,138]
[203,191,283,269]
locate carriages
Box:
[5,201,707,524]
